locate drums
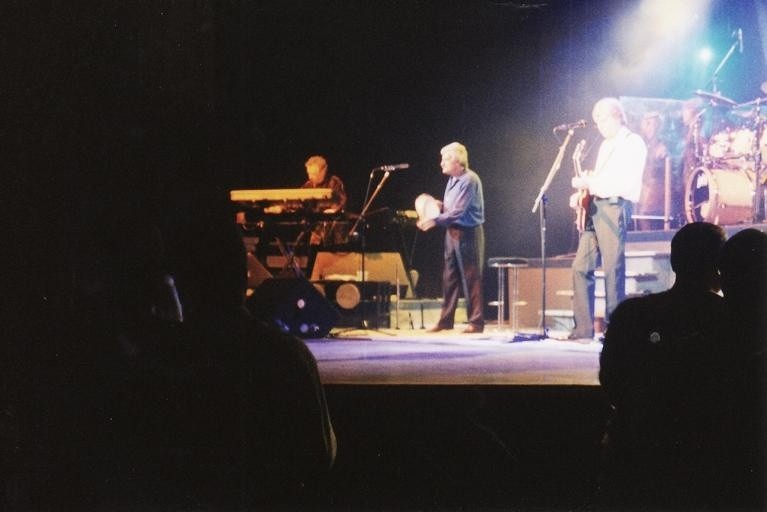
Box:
[731,125,756,157]
[709,128,730,159]
[685,166,756,226]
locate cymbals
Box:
[693,91,737,106]
[732,105,767,119]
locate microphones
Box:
[557,119,587,131]
[380,162,409,171]
[737,28,743,53]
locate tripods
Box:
[734,104,767,226]
[336,171,399,337]
[532,129,580,343]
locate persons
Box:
[599,221,767,512]
[654,96,704,227]
[565,97,648,341]
[300,156,348,215]
[1,220,338,512]
[416,142,486,333]
[630,110,671,232]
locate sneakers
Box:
[427,325,482,333]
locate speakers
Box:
[324,279,392,328]
[247,276,341,339]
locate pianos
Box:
[230,188,336,203]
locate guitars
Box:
[573,139,590,235]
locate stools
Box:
[487,257,529,338]
